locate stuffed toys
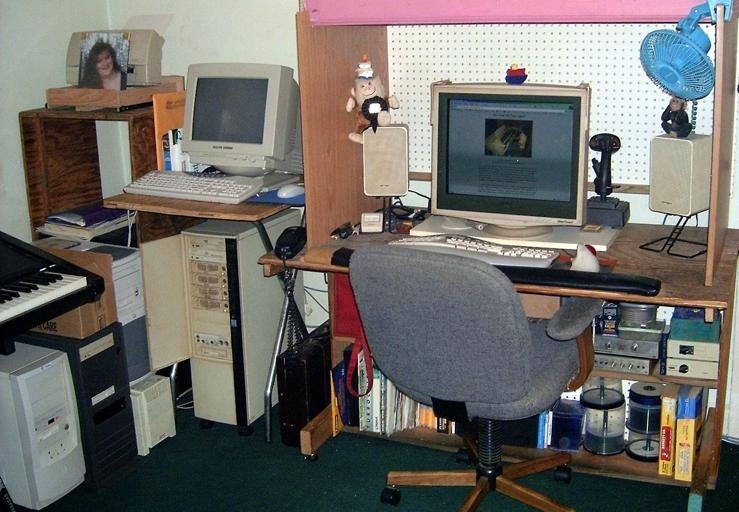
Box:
[659,95,693,139]
[346,75,403,145]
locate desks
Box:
[103,173,304,443]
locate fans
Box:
[639,1,734,101]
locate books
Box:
[336,345,457,439]
[674,385,709,482]
[160,126,221,173]
[658,382,680,476]
[37,200,137,244]
[536,407,553,449]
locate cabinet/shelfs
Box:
[256,1,739,512]
[17,105,207,246]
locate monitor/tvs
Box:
[180,63,303,193]
[430,79,593,241]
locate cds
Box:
[583,382,667,458]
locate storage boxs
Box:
[30,241,118,340]
[123,315,150,382]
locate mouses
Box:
[276,183,305,198]
[571,240,600,272]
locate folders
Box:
[44,200,124,228]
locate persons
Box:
[81,40,128,91]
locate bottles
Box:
[579,372,659,463]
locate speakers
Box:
[648,132,713,218]
[362,124,410,197]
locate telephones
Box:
[275,226,306,260]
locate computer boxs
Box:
[0,341,86,511]
[30,235,152,389]
[15,321,139,491]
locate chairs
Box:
[348,243,604,512]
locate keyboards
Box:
[387,234,561,270]
[123,169,264,205]
